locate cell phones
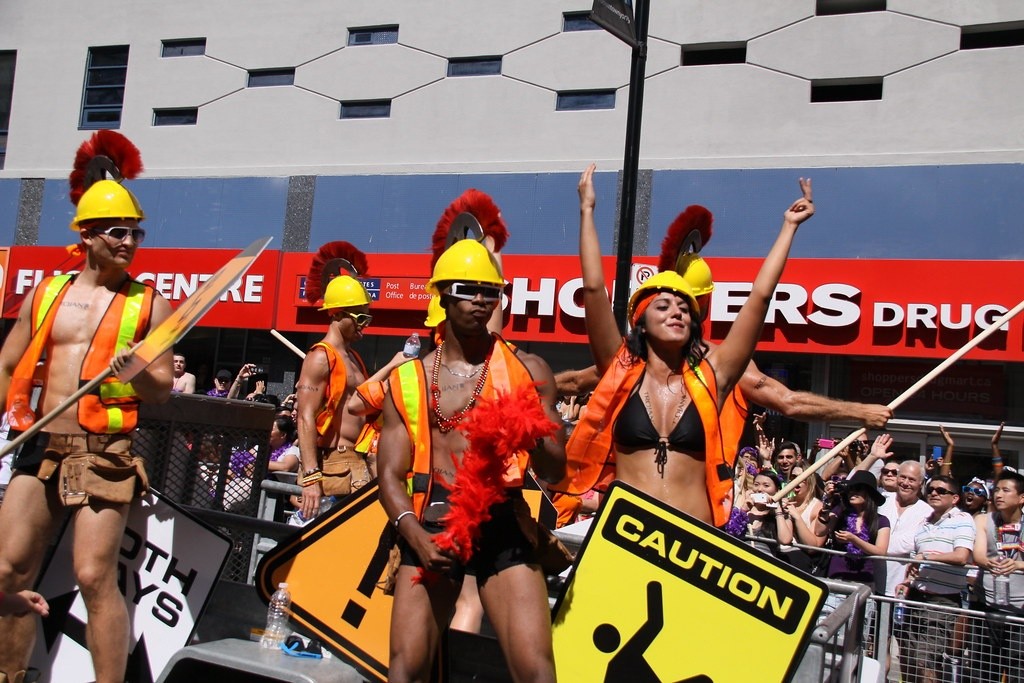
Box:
[750,493,768,502]
[818,439,835,449]
[933,446,941,462]
[250,366,264,373]
[834,480,849,490]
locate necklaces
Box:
[431,341,490,430]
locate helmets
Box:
[68,128,146,232]
[304,240,372,312]
[424,188,510,296]
[626,269,701,332]
[656,205,715,298]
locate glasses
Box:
[215,378,232,382]
[87,226,146,245]
[926,486,955,496]
[962,485,986,497]
[881,467,897,476]
[339,310,373,328]
[440,282,503,303]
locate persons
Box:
[552,251,894,528]
[0,590,49,617]
[171,353,300,484]
[568,489,599,524]
[348,212,502,634]
[0,179,175,683]
[376,239,567,683]
[547,162,814,528]
[297,275,378,517]
[728,412,1024,683]
[555,395,580,423]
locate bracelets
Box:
[235,379,242,384]
[923,553,928,560]
[774,512,782,516]
[395,511,417,527]
[302,467,322,487]
[817,516,830,524]
[943,462,952,465]
[992,457,1002,466]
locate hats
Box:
[216,370,232,379]
[846,470,886,507]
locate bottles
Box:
[260,582,290,650]
[893,590,905,623]
[293,496,337,525]
[403,334,421,358]
[993,552,1009,606]
